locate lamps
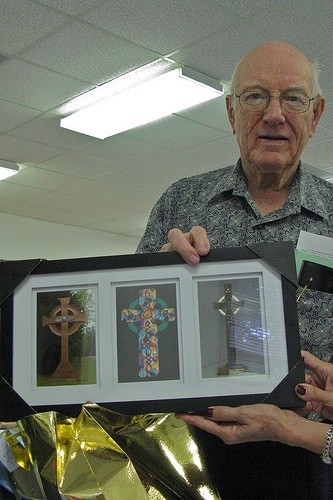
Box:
[58,65,224,141]
[1,161,19,181]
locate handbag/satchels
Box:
[0,399,224,500]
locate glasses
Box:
[233,91,320,115]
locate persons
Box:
[134,41,333,500]
[175,349,333,466]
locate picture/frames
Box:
[1,243,308,420]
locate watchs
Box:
[319,425,333,465]
